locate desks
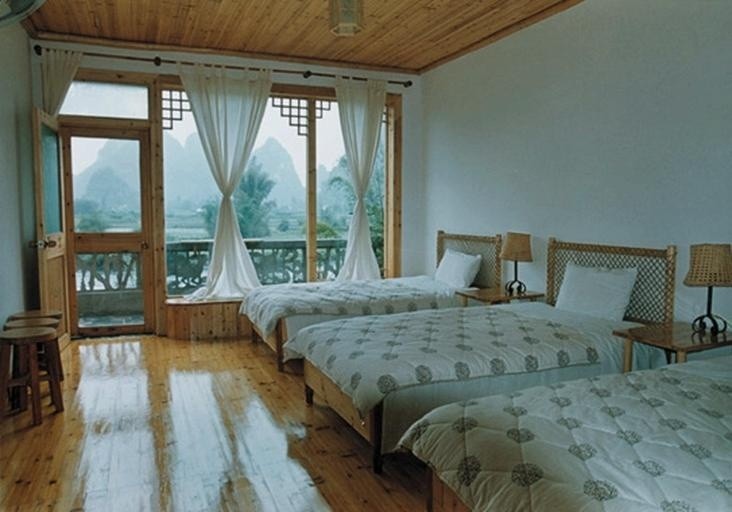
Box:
[454,285,545,307]
[609,321,731,371]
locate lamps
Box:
[683,243,732,334]
[326,1,365,38]
[499,232,533,293]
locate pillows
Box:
[434,248,483,288]
[554,260,639,320]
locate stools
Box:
[3,317,63,411]
[9,309,64,382]
[1,327,65,424]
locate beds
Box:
[238,228,503,371]
[280,236,678,472]
[395,352,730,509]
